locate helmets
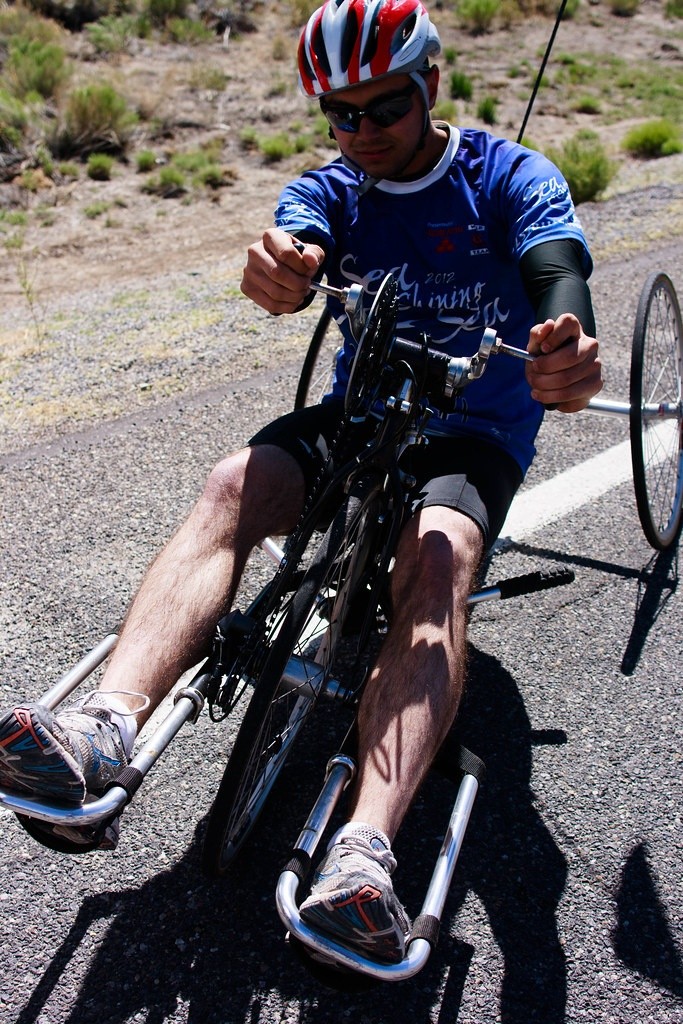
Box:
[296,1,442,101]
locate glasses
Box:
[319,71,424,134]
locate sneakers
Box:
[285,840,406,979]
[0,701,130,852]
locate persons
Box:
[0,2,603,980]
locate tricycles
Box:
[1,247,680,981]
